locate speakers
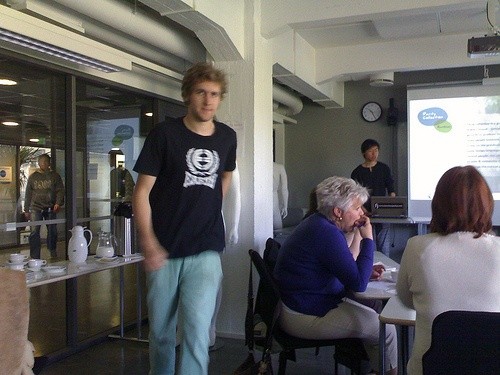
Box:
[387,108,398,126]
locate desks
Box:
[353,250,401,309]
[379,297,417,375]
[368,217,432,257]
[23,255,146,364]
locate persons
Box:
[273,139,500,375]
[132,63,237,375]
[107,150,136,258]
[272,163,289,231]
[24,154,65,259]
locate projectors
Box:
[468,35,500,58]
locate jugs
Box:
[67,225,93,264]
[96,231,118,258]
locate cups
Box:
[27,259,46,269]
[9,253,28,264]
[8,262,24,271]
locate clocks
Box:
[361,101,382,122]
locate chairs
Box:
[0,267,36,375]
[248,249,370,375]
[421,309,500,375]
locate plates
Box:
[42,265,66,273]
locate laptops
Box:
[370,196,408,219]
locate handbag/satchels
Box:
[234,353,273,375]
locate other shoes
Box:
[48,250,57,259]
